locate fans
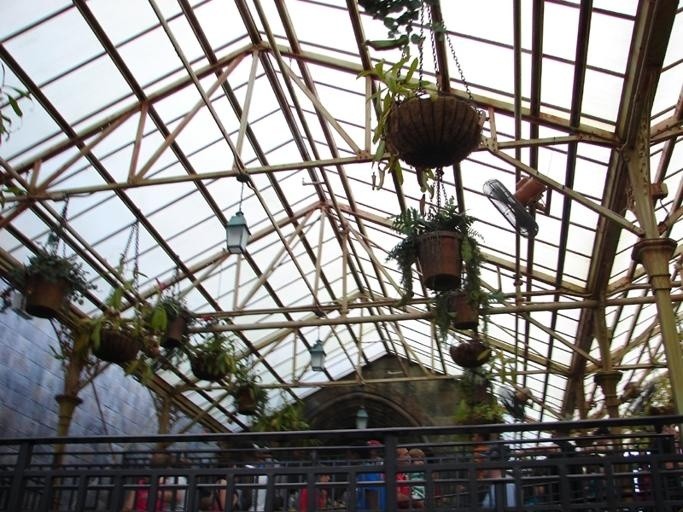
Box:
[482,175,546,239]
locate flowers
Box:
[155,277,216,323]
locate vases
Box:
[165,316,187,347]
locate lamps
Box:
[225,179,251,255]
[310,312,327,369]
[355,385,368,429]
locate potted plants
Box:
[356,57,480,193]
[385,195,521,407]
[1,244,155,388]
[189,337,310,440]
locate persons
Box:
[123,423,682,511]
